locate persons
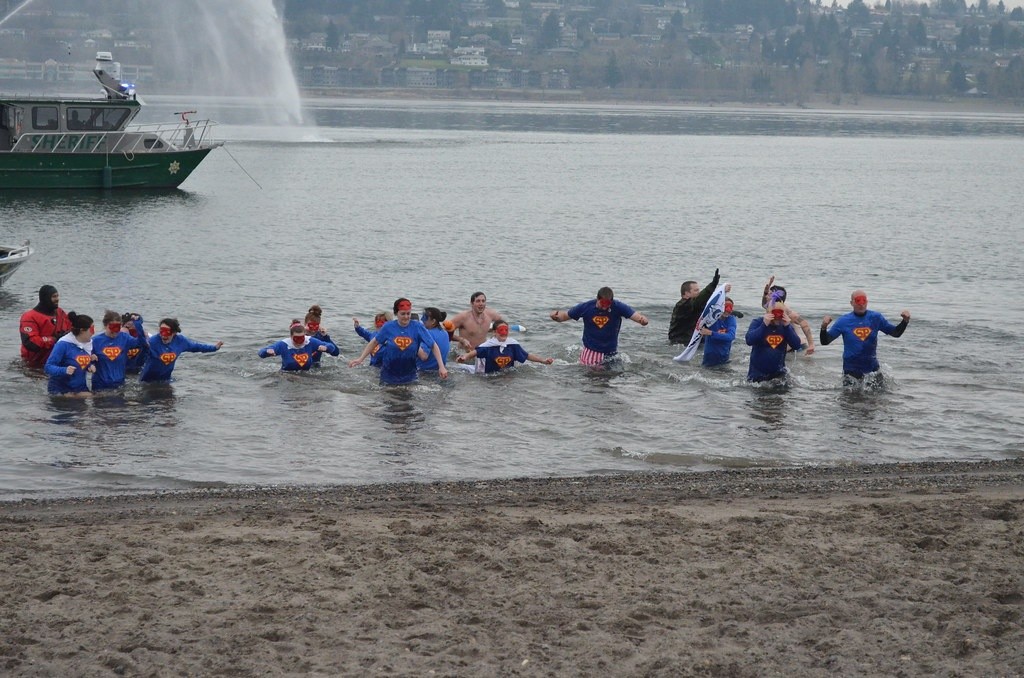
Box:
[352,311,393,367]
[450,291,502,353]
[762,276,814,355]
[20,285,72,367]
[140,318,223,383]
[416,307,449,370]
[745,302,801,382]
[455,320,553,374]
[699,297,737,367]
[258,319,335,371]
[120,312,152,372]
[668,268,720,347]
[303,305,339,362]
[44,311,96,394]
[550,287,648,365]
[820,289,910,386]
[348,297,448,386]
[91,309,146,390]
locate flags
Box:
[672,284,725,361]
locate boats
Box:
[0,239,35,287]
[0,51,226,187]
